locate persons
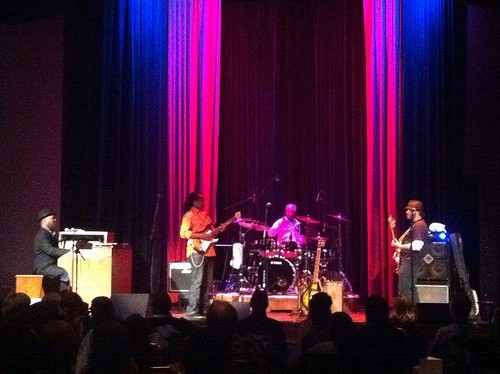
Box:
[33,207,69,285]
[0,275,500,374]
[179,191,227,318]
[391,200,429,313]
[269,204,301,242]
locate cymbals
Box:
[327,213,352,222]
[237,219,271,232]
[295,216,321,223]
[306,236,329,240]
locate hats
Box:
[405,200,423,211]
[190,192,202,199]
[286,204,297,212]
[36,207,55,222]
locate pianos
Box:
[57,242,133,309]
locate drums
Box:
[255,256,300,295]
[280,241,298,258]
[258,238,281,258]
[309,249,335,260]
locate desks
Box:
[56,243,133,310]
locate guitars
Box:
[388,216,401,275]
[301,240,325,311]
[189,211,240,253]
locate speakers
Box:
[111,293,153,320]
[414,281,450,322]
[169,263,192,293]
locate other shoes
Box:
[186,313,207,320]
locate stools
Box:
[14,274,45,299]
